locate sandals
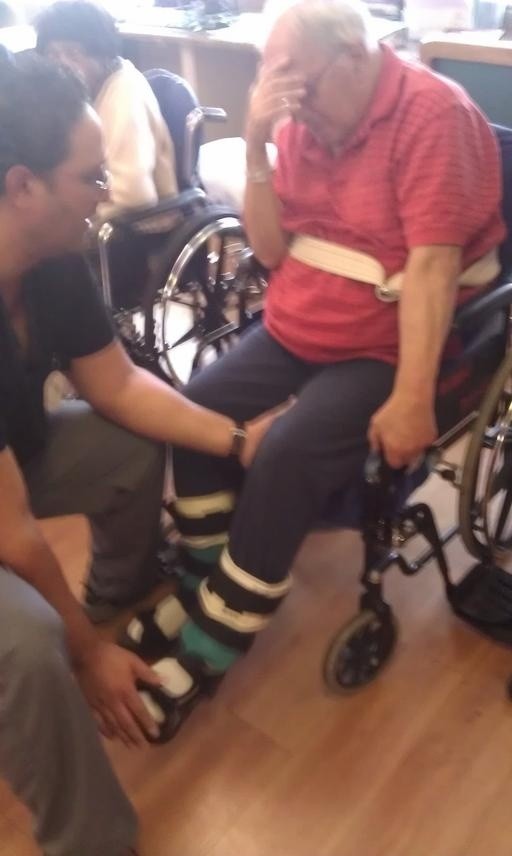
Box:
[136,638,226,744]
[118,585,196,658]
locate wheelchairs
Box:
[71,66,276,409]
[131,118,511,697]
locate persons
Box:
[0,51,297,856]
[106,0,510,747]
[31,0,186,416]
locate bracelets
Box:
[226,417,248,464]
